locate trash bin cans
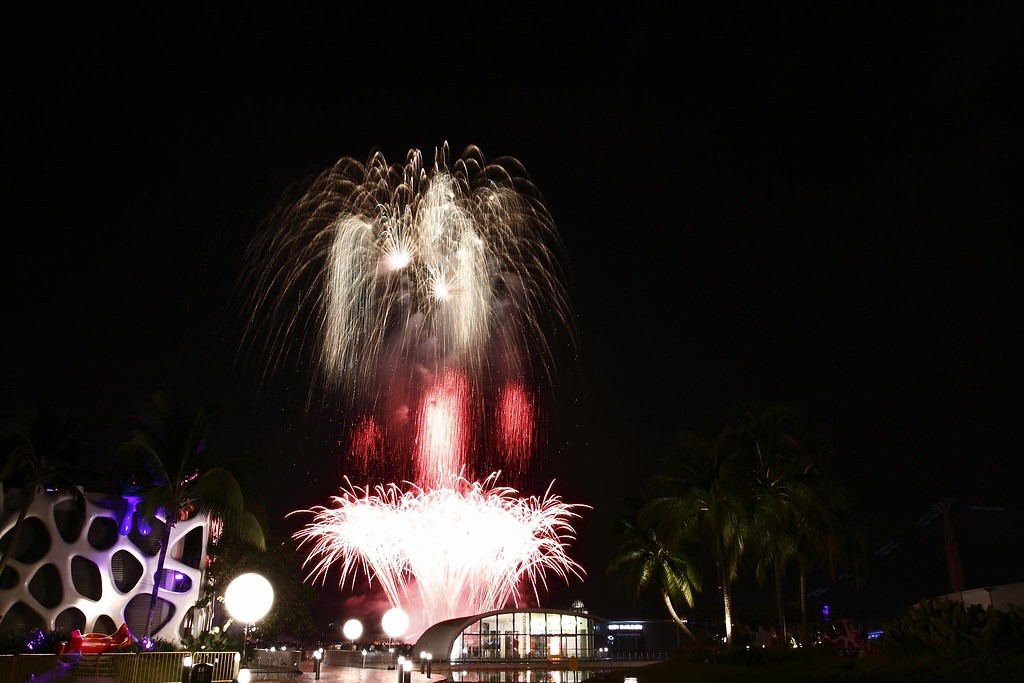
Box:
[182,662,213,683]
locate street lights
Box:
[144,469,200,652]
[0,483,61,575]
